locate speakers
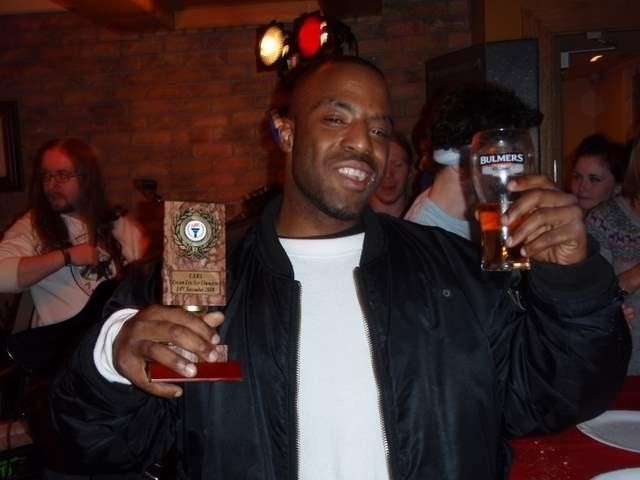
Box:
[425,38,540,134]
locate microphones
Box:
[106,203,129,269]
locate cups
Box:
[469,126,533,272]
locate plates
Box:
[575,408,640,455]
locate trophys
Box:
[146,202,241,382]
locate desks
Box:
[503,372,639,479]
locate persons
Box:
[22,45,632,480]
[1,135,149,328]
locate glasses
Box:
[40,168,76,183]
